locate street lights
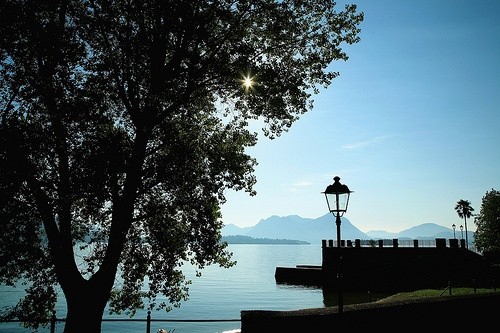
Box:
[460,225,464,239]
[323,176,354,312]
[452,224,456,239]
